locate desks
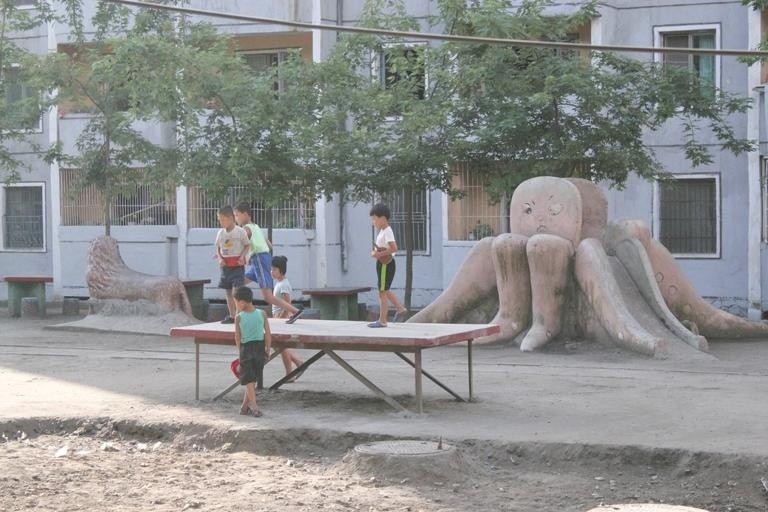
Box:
[180,278,213,320]
[4,273,54,320]
[170,313,500,416]
[301,284,376,320]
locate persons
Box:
[232,285,273,418]
[267,253,307,383]
[365,203,407,328]
[210,200,303,328]
[214,202,252,325]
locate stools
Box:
[357,303,368,320]
[63,297,79,314]
[189,298,210,321]
[20,297,41,318]
[300,308,321,320]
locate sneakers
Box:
[221,317,235,324]
[239,406,263,417]
[286,365,308,384]
[284,309,304,324]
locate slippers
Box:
[393,309,406,323]
[367,321,387,328]
